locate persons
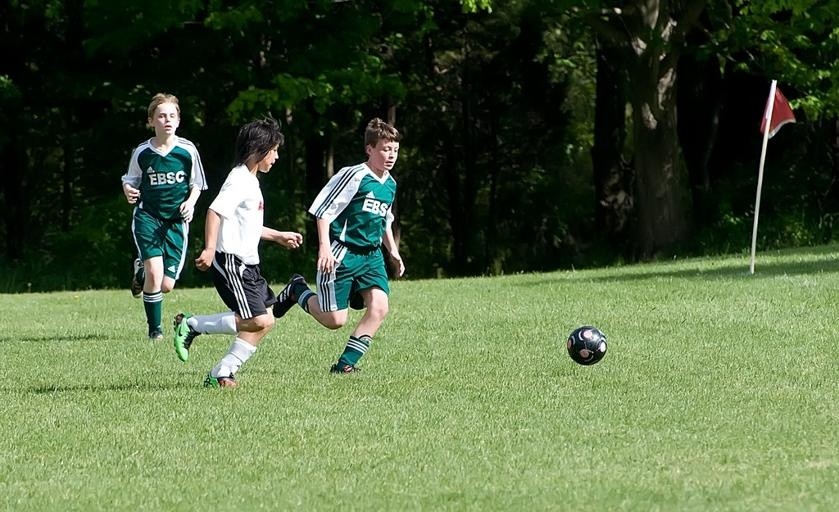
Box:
[274,118,405,375]
[173,120,303,388]
[121,93,209,340]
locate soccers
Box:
[567,325,607,365]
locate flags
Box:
[760,80,797,139]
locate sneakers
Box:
[330,363,361,374]
[172,313,202,362]
[204,370,238,389]
[273,272,307,318]
[131,259,145,298]
[149,326,165,340]
